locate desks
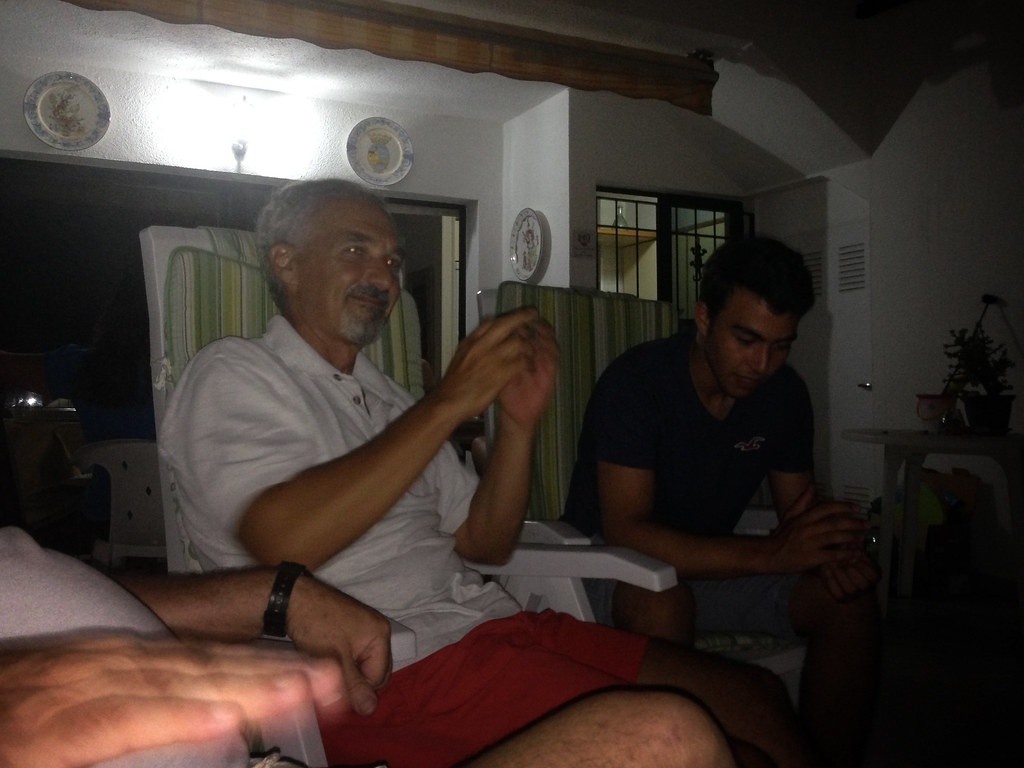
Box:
[841,427,1024,619]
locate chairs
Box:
[70,440,167,571]
[471,284,882,672]
[138,223,676,623]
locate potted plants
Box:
[915,325,1016,436]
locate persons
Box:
[0,523,735,768]
[155,178,807,768]
[560,235,883,768]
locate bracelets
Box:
[263,561,313,638]
